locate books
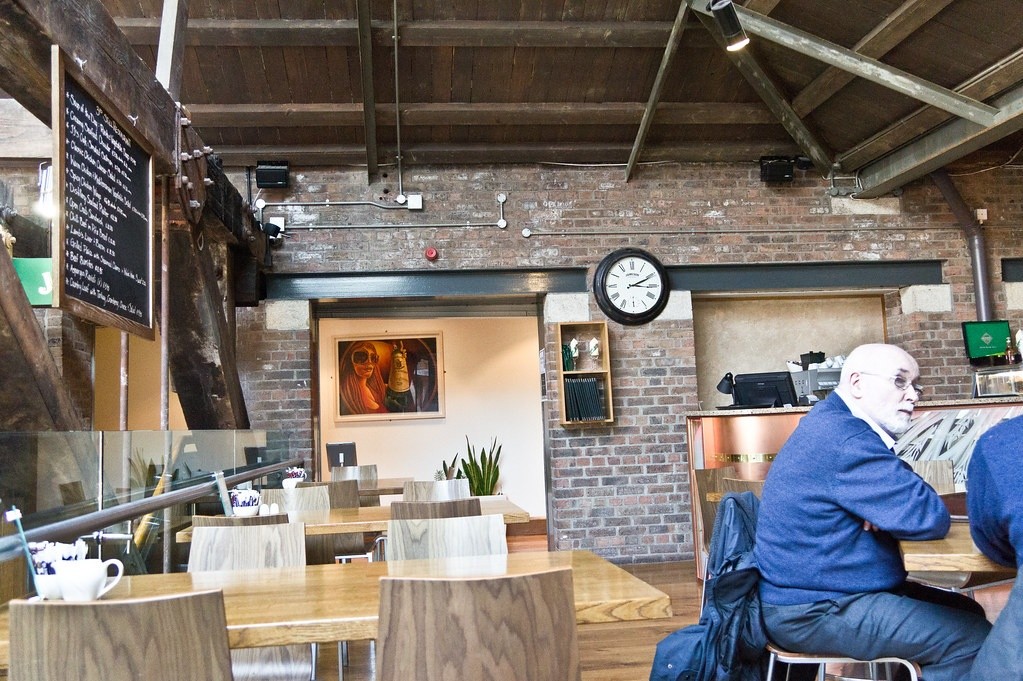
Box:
[565,378,605,422]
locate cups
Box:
[259,503,279,516]
[35,575,64,599]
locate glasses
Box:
[852,371,924,397]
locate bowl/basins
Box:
[233,506,258,517]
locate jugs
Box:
[51,559,124,602]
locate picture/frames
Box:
[332,330,446,423]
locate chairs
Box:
[7,464,580,681]
[690,461,1016,681]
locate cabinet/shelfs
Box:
[555,320,614,425]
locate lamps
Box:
[758,156,794,182]
[716,371,741,410]
[255,160,290,188]
[709,0,750,52]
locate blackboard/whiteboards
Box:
[51,44,155,342]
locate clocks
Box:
[593,247,671,326]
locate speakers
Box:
[255,161,289,188]
[759,156,792,183]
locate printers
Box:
[792,351,842,407]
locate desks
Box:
[899,523,1015,573]
[358,478,413,496]
[175,500,529,543]
[0,548,674,681]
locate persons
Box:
[752,343,994,681]
[966,414,1023,681]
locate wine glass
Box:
[590,342,600,371]
[570,345,580,371]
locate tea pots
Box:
[282,479,297,489]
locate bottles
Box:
[1005,337,1015,365]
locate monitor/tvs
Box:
[961,320,1012,367]
[735,373,798,408]
